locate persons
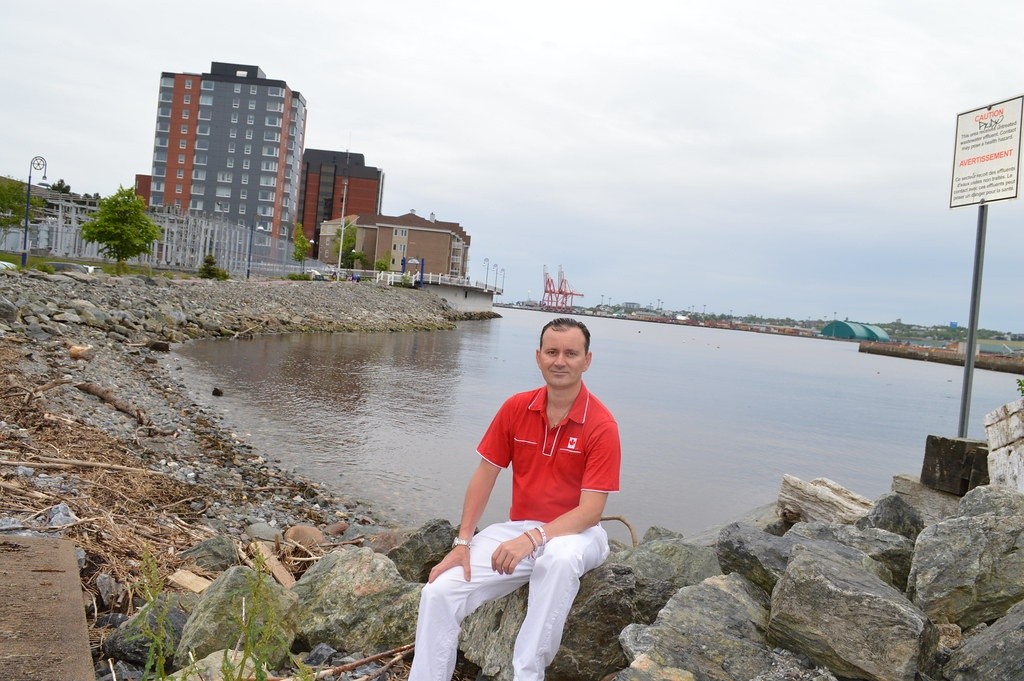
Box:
[332,273,337,281]
[347,274,360,282]
[408,319,621,681]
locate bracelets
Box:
[524,531,538,560]
[535,526,546,546]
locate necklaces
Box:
[549,407,569,425]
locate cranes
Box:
[540,264,584,311]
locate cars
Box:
[31,261,88,275]
[0,261,17,271]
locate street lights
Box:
[492,264,498,295]
[245,212,265,280]
[499,268,505,296]
[345,248,356,277]
[482,257,489,293]
[21,156,51,268]
[299,238,315,273]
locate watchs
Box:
[451,537,471,550]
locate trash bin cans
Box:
[307,271,315,281]
